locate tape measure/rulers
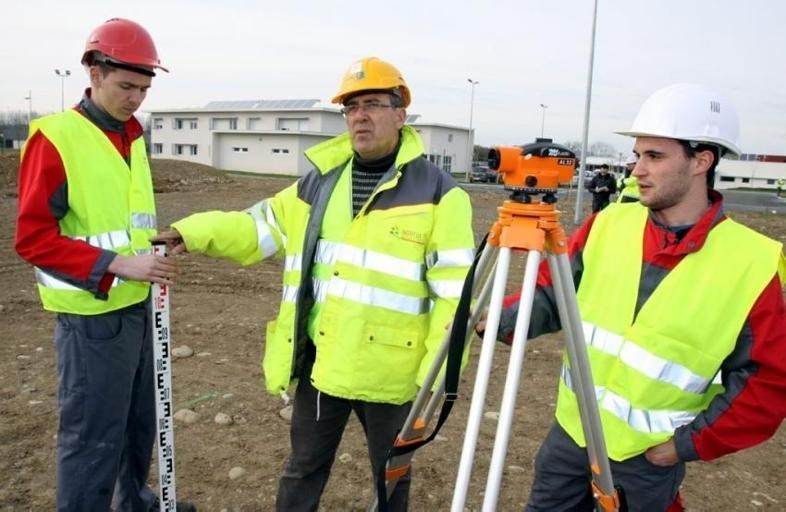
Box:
[150,241,176,512]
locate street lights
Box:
[55,69,71,112]
[465,79,480,182]
[541,103,548,139]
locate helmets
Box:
[331,57,411,108]
[81,18,169,77]
[612,84,743,159]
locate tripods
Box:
[370,204,630,512]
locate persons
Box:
[615,156,639,202]
[446,79,786,512]
[587,164,617,214]
[148,58,478,512]
[14,17,198,512]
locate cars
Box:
[559,169,623,190]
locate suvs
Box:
[470,166,496,184]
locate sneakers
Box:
[149,496,196,512]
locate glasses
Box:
[340,103,388,114]
